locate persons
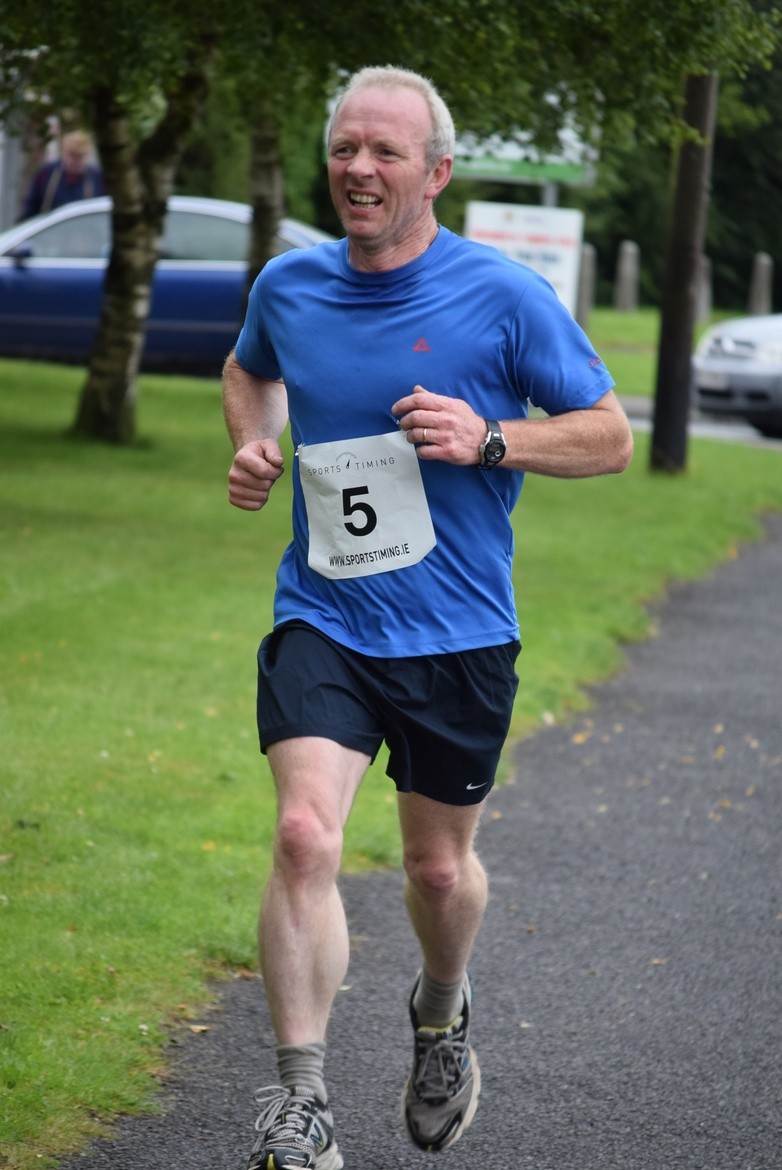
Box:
[226,65,632,1169]
[13,128,107,227]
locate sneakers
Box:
[247,1085,345,1170]
[403,968,482,1153]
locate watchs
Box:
[479,419,506,470]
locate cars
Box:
[689,314,782,441]
[0,193,343,374]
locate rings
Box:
[423,427,427,443]
